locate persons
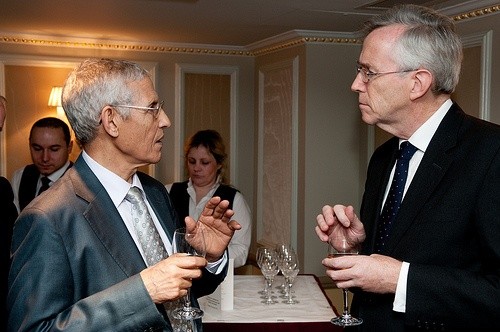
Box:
[12,58,242,332]
[164,130,252,268]
[0,94,19,332]
[315,3,500,332]
[12,117,75,214]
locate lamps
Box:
[47,86,66,115]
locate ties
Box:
[125,186,192,332]
[37,176,52,196]
[372,141,418,253]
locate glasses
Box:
[99,99,164,125]
[355,65,418,84]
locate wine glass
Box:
[171,227,205,320]
[327,234,363,325]
[257,241,300,305]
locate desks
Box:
[199,274,352,332]
